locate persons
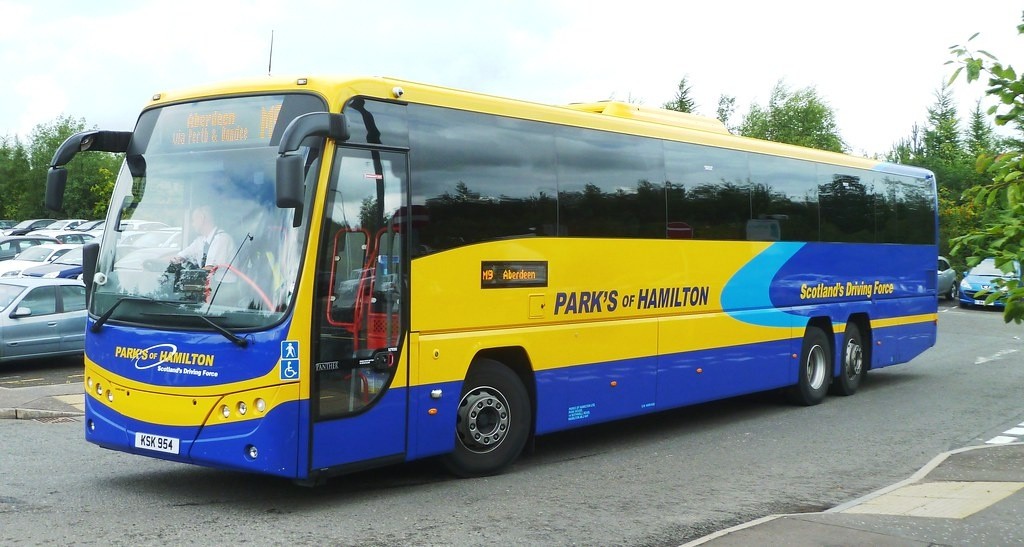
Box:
[158,206,239,305]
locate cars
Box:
[957,256,1024,310]
[937,255,958,302]
[0,217,184,368]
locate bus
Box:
[45,75,939,476]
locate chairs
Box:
[6,243,16,257]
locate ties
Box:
[200,229,218,268]
